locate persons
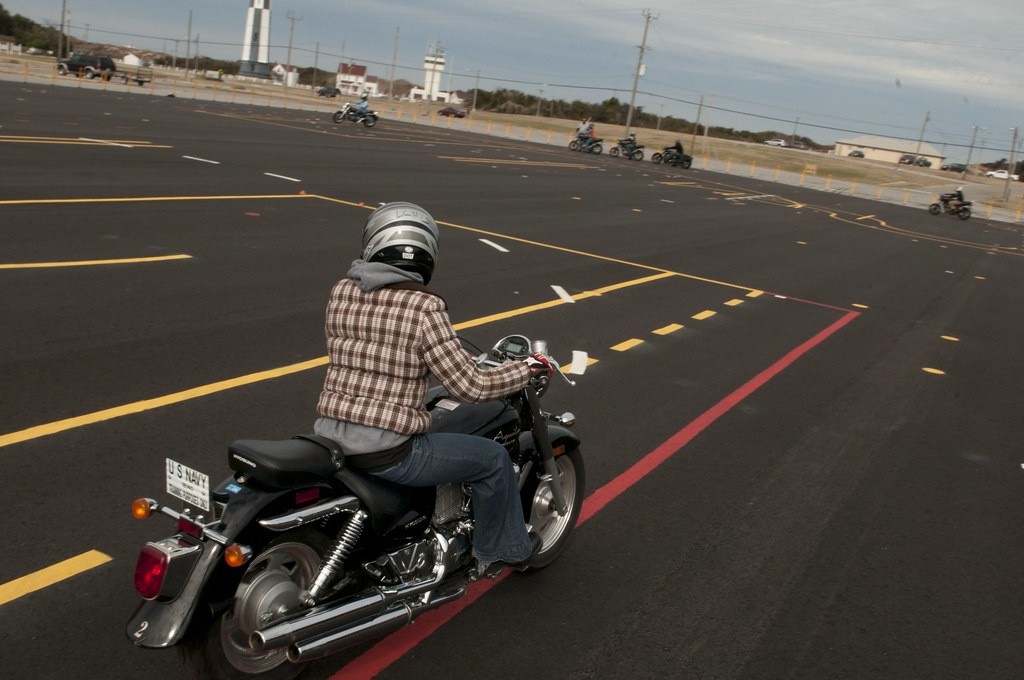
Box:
[620,132,636,159]
[576,116,596,154]
[349,93,369,123]
[945,186,964,215]
[315,201,554,577]
[666,138,683,166]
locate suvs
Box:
[56,52,116,81]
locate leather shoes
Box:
[474,531,543,569]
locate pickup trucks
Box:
[985,170,1020,181]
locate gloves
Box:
[527,353,553,379]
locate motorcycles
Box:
[929,195,973,220]
[125,336,587,680]
[609,139,645,161]
[651,147,693,169]
[333,103,378,127]
[569,132,605,154]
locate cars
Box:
[787,141,806,149]
[437,107,467,118]
[765,139,785,147]
[316,87,341,98]
[942,163,966,173]
[898,154,931,167]
[848,150,864,158]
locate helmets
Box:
[676,140,680,143]
[362,94,367,99]
[956,187,963,191]
[361,201,440,286]
[631,133,635,136]
